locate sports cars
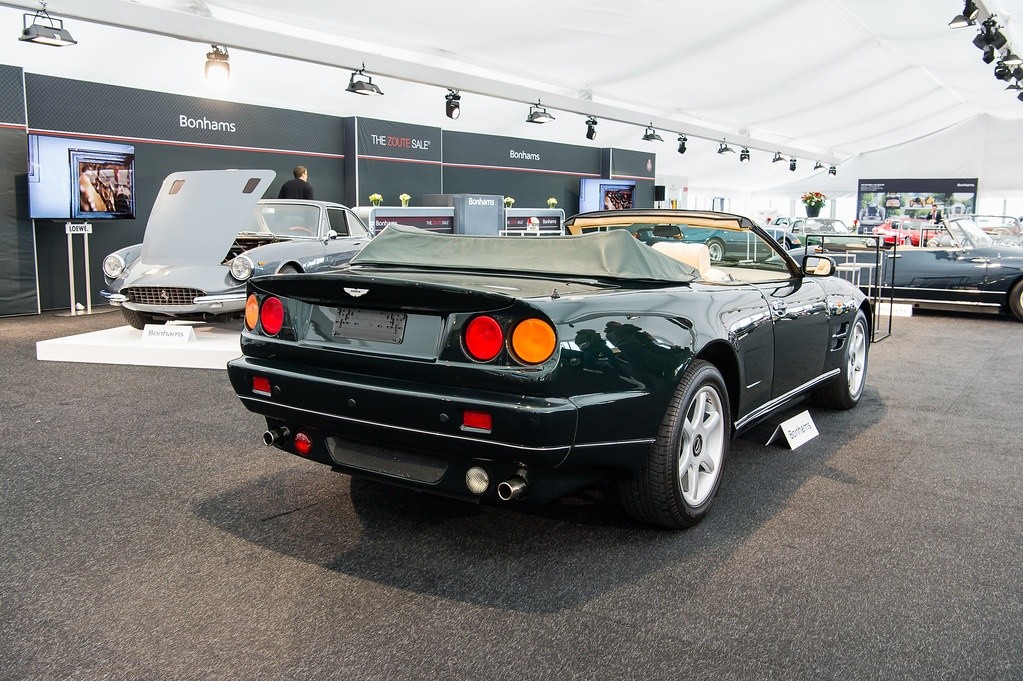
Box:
[227,208,875,529]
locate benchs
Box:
[891,244,915,250]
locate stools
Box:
[814,253,862,289]
[838,250,884,335]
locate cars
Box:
[854,217,885,233]
[790,218,876,249]
[635,222,802,263]
[871,215,941,247]
[100,165,377,329]
[982,216,1023,235]
[765,217,801,225]
[767,214,1023,320]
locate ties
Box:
[932,210,936,219]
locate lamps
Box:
[813,161,827,171]
[205,44,232,83]
[716,140,736,154]
[739,146,751,165]
[677,134,688,154]
[525,104,555,125]
[18,13,77,48]
[948,0,1023,102]
[772,152,786,164]
[585,115,597,140]
[789,156,797,171]
[445,88,461,120]
[828,164,837,177]
[641,126,664,143]
[344,72,385,96]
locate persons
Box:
[278,165,314,200]
[926,205,942,224]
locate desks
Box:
[500,230,565,236]
[804,233,898,343]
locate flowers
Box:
[852,219,859,232]
[504,196,515,207]
[546,196,559,206]
[398,192,412,207]
[527,217,540,229]
[800,191,829,207]
[368,192,384,206]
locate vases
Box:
[373,200,381,206]
[853,225,858,234]
[550,203,555,208]
[402,200,409,207]
[527,223,539,231]
[506,202,512,208]
[805,206,822,217]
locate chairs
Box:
[651,241,730,281]
